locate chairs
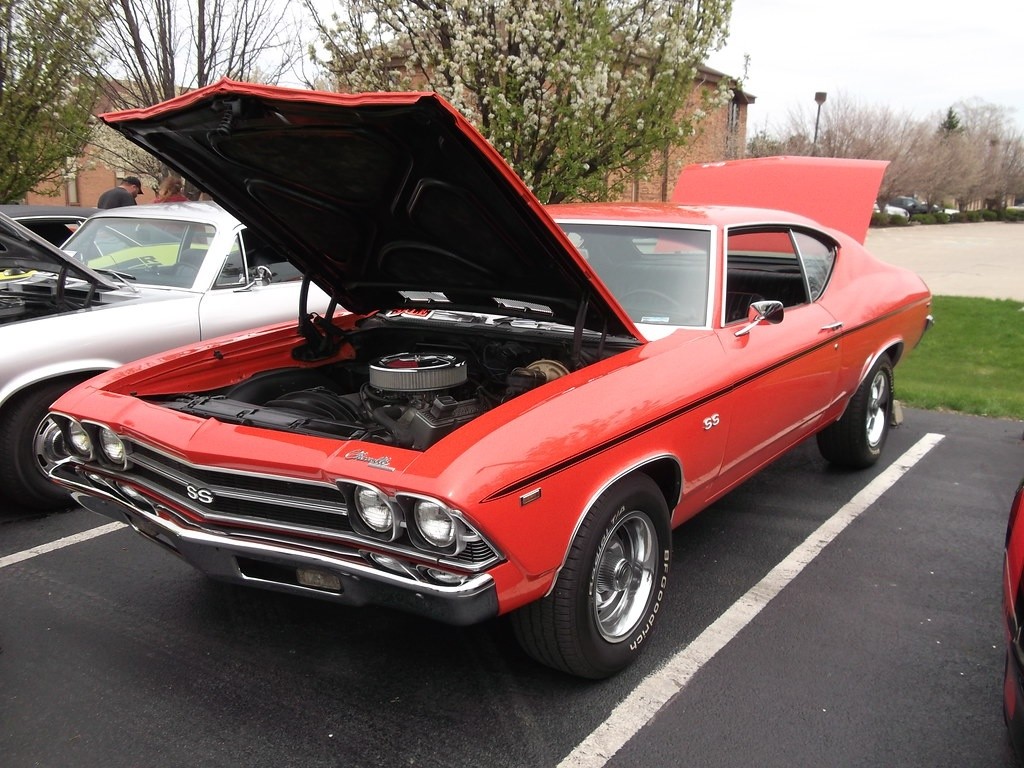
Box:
[174,248,207,277]
[725,290,764,323]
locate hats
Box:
[124,176,144,195]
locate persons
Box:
[97,176,144,210]
[152,176,191,203]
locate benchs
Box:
[613,263,808,307]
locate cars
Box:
[0,199,344,511]
[2,204,184,265]
[873,195,960,223]
[42,75,937,681]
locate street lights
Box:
[811,91,827,157]
